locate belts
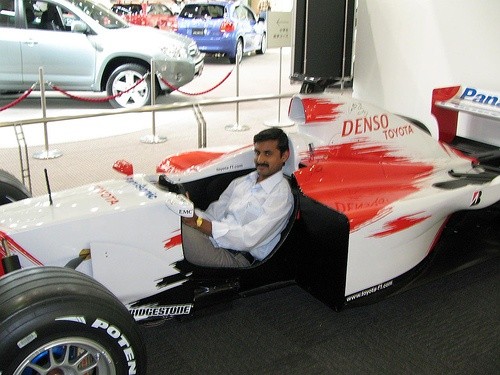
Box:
[228,248,258,266]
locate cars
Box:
[172,2,269,64]
[0,1,205,109]
[103,1,181,37]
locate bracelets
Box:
[196,216,203,230]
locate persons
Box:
[181,128,294,271]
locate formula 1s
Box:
[0,85,500,375]
